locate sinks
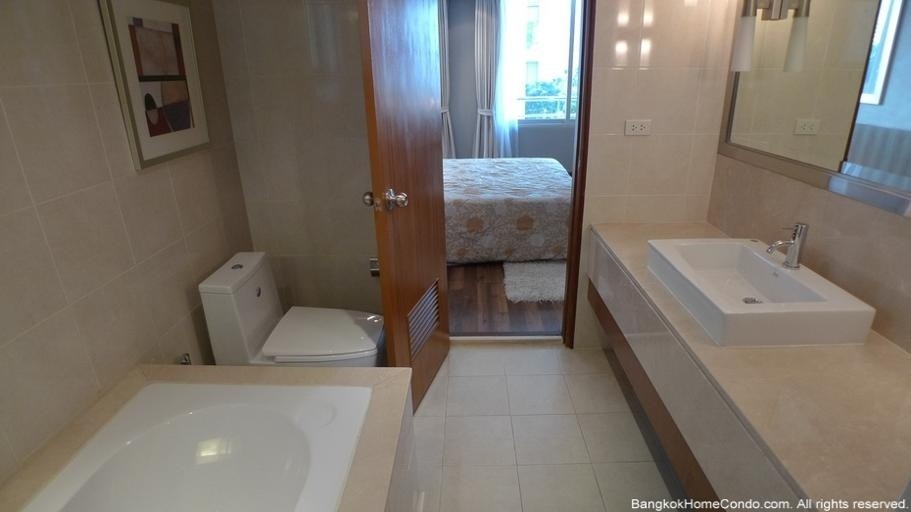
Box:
[24,381,373,511]
[646,238,877,347]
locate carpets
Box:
[503,259,567,304]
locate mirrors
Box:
[716,0,911,218]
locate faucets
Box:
[766,223,809,268]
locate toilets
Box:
[198,252,386,367]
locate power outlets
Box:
[794,118,821,136]
[624,119,651,137]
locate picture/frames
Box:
[98,0,215,170]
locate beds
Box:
[442,158,576,264]
